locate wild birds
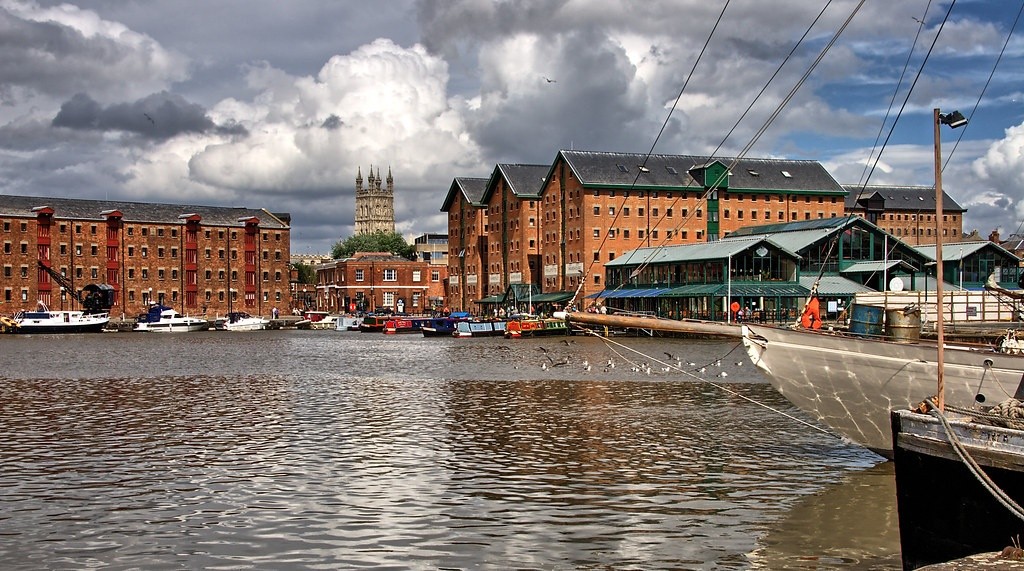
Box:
[541,74,558,85]
[630,351,743,379]
[558,338,576,347]
[539,346,616,373]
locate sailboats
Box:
[550,1,1023,464]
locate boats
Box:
[10,299,112,333]
[890,395,1024,571]
[215,312,272,332]
[131,303,207,333]
[360,309,569,338]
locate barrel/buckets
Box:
[884,309,921,344]
[848,304,885,340]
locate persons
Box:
[730,302,766,322]
[432,303,537,319]
[288,307,305,316]
[571,302,610,317]
